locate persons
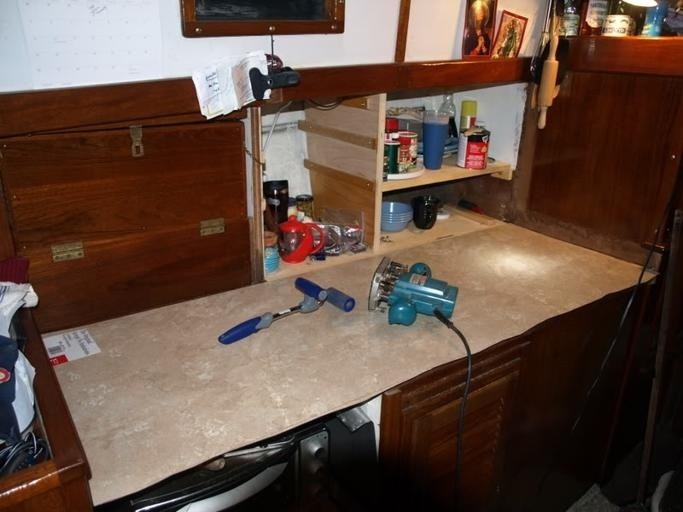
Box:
[464,2,491,55]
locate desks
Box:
[42,226,658,505]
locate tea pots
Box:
[275,215,325,263]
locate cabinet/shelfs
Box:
[378,83,529,246]
[2,127,254,331]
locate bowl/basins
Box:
[380,200,414,232]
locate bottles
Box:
[438,92,478,138]
[558,1,669,37]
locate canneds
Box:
[383,140,400,181]
[397,131,418,174]
[456,127,491,170]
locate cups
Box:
[422,109,449,170]
[409,196,439,230]
